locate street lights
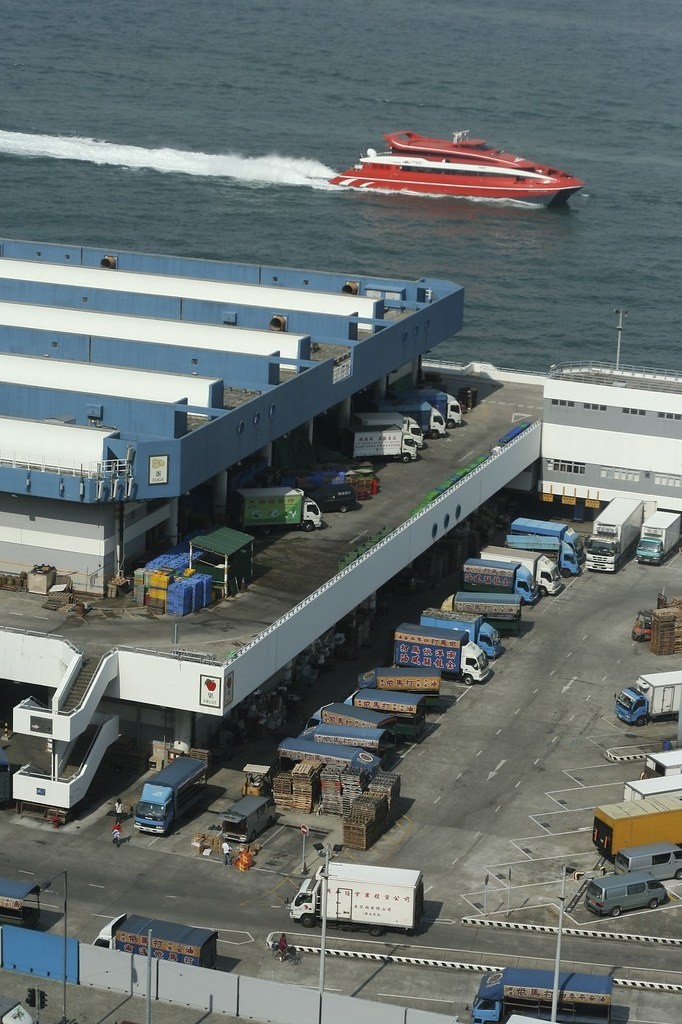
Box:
[550,865,576,1022]
[41,868,67,1023]
[313,842,345,1024]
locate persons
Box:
[584,534,591,549]
[637,700,642,706]
[222,839,233,866]
[663,734,672,751]
[112,820,122,847]
[298,897,304,904]
[279,933,288,962]
[115,798,123,822]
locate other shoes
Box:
[278,956,282,962]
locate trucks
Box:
[276,497,681,781]
[227,387,463,533]
[0,994,35,1024]
[465,967,614,1024]
[289,861,424,938]
[91,912,218,970]
[129,756,207,835]
[614,670,682,727]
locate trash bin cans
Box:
[458,387,477,409]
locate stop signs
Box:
[301,824,309,836]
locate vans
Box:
[584,872,668,917]
[217,794,276,843]
[613,841,682,881]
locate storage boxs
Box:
[273,759,401,851]
[134,531,212,616]
[651,597,682,655]
[345,473,377,500]
[190,748,212,759]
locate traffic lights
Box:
[26,988,48,1009]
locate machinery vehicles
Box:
[241,764,273,798]
[632,609,654,643]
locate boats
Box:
[327,131,586,209]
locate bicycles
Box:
[272,946,300,965]
